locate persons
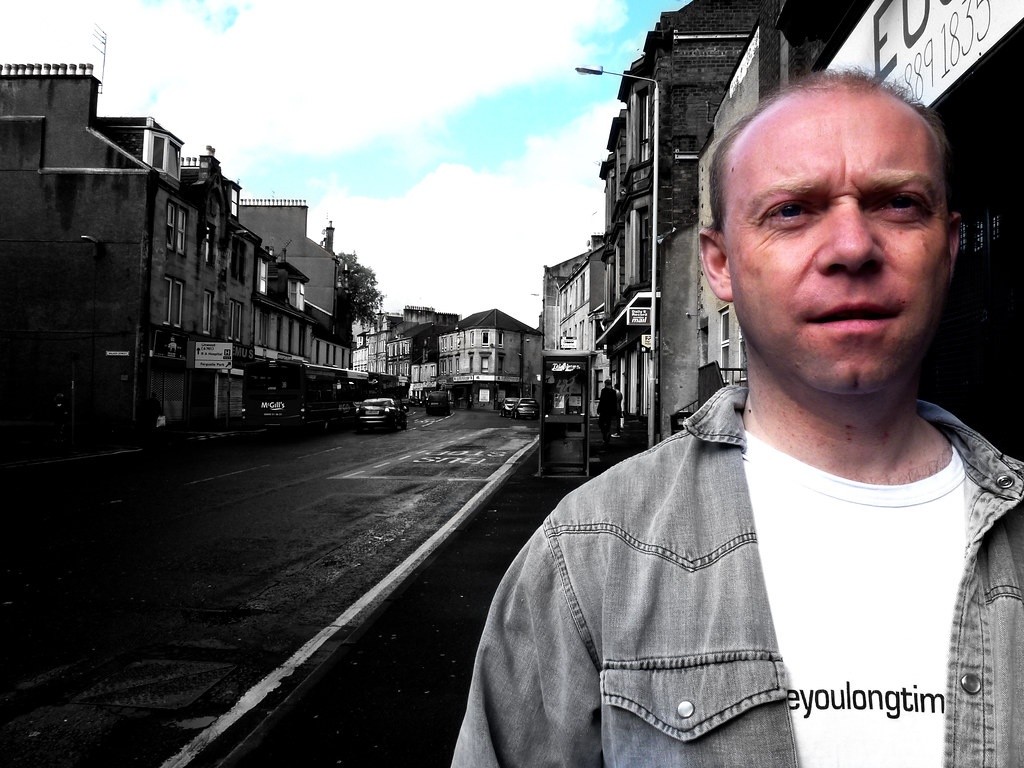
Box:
[456,63,1024,767]
[597,379,618,445]
[47,391,163,448]
[611,383,623,438]
[466,393,473,410]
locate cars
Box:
[499,397,520,416]
[510,397,540,419]
[355,398,407,435]
[425,391,451,415]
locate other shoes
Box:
[611,433,620,437]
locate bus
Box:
[242,358,401,442]
